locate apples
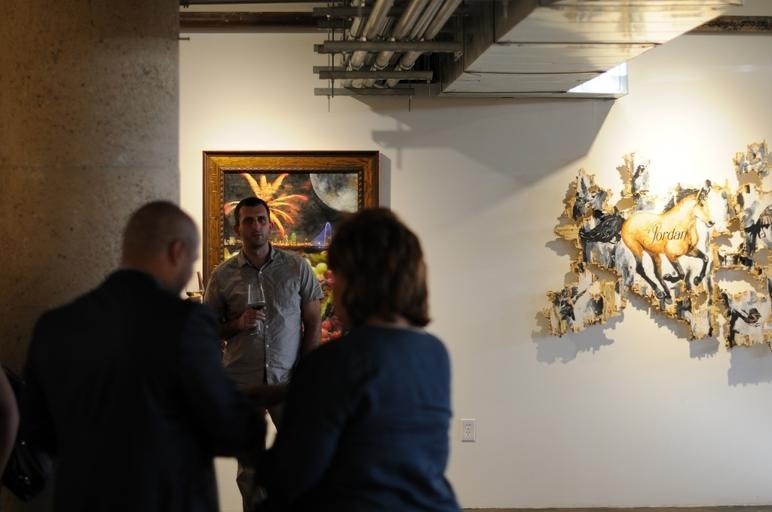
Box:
[322,320,341,343]
[304,257,336,287]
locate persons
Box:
[20,193,269,511]
[250,206,461,512]
[201,194,328,511]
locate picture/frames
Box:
[202,149,379,340]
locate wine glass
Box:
[247,282,265,337]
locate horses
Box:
[579,184,717,306]
